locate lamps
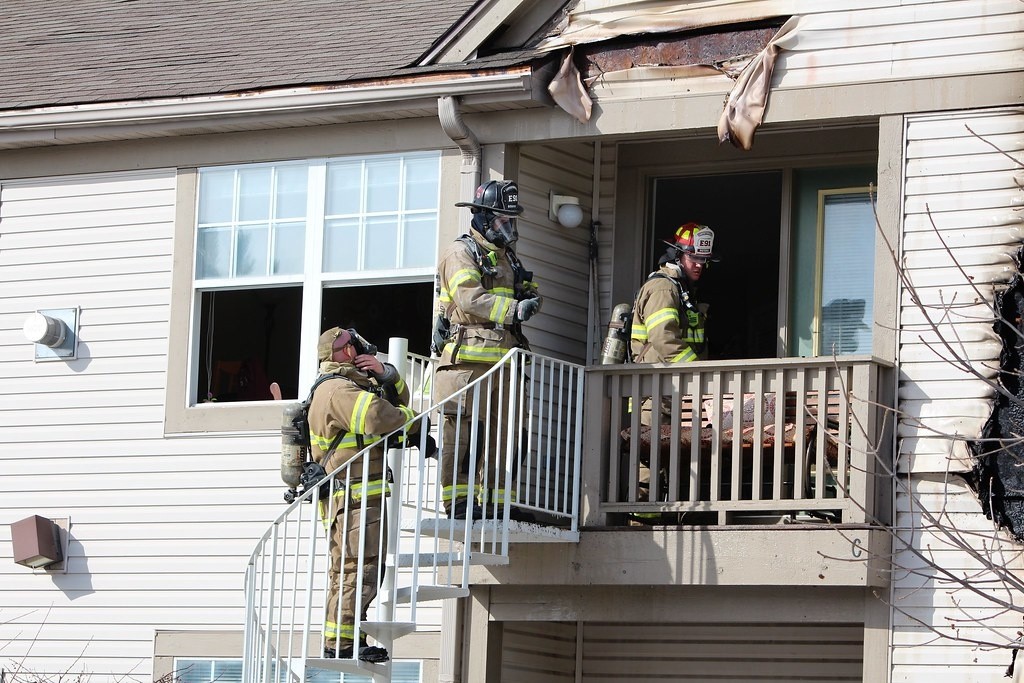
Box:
[21,304,81,362]
[12,514,71,570]
[549,191,585,229]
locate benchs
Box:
[620,389,854,523]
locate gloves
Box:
[516,297,543,321]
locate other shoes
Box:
[324,643,389,664]
[446,501,484,519]
[488,507,536,524]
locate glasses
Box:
[681,253,707,265]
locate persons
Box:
[619,218,717,529]
[437,179,542,527]
[299,327,433,664]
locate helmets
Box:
[454,180,524,216]
[660,223,714,256]
